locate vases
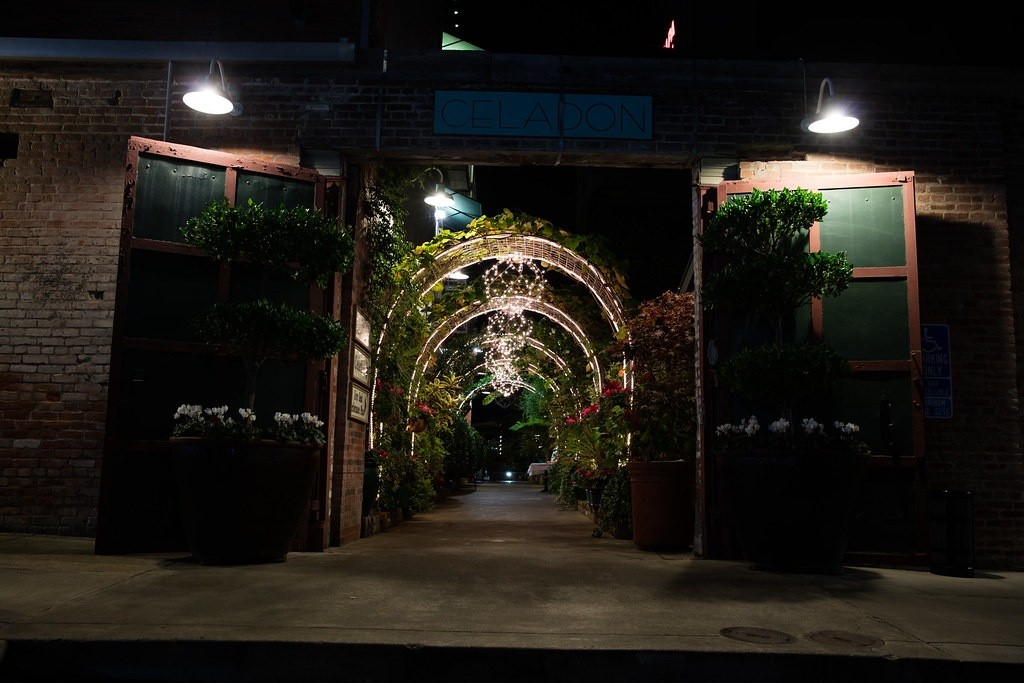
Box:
[574,488,602,510]
[168,434,322,565]
[625,460,695,548]
[744,453,855,571]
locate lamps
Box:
[182,59,245,118]
[449,268,469,280]
[801,77,861,134]
[407,167,455,207]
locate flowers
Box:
[697,189,872,454]
[553,383,625,486]
[170,198,358,445]
[619,365,669,457]
